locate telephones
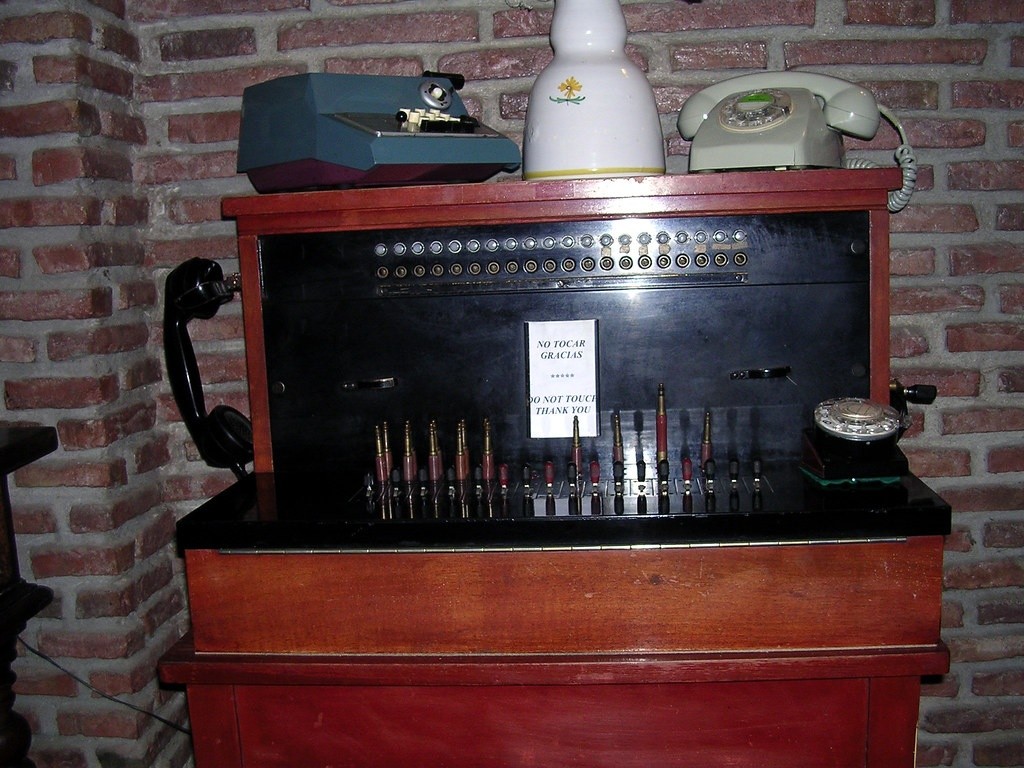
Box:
[677,70,880,172]
[163,257,252,469]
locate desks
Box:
[155,631,950,768]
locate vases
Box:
[522,0,664,180]
